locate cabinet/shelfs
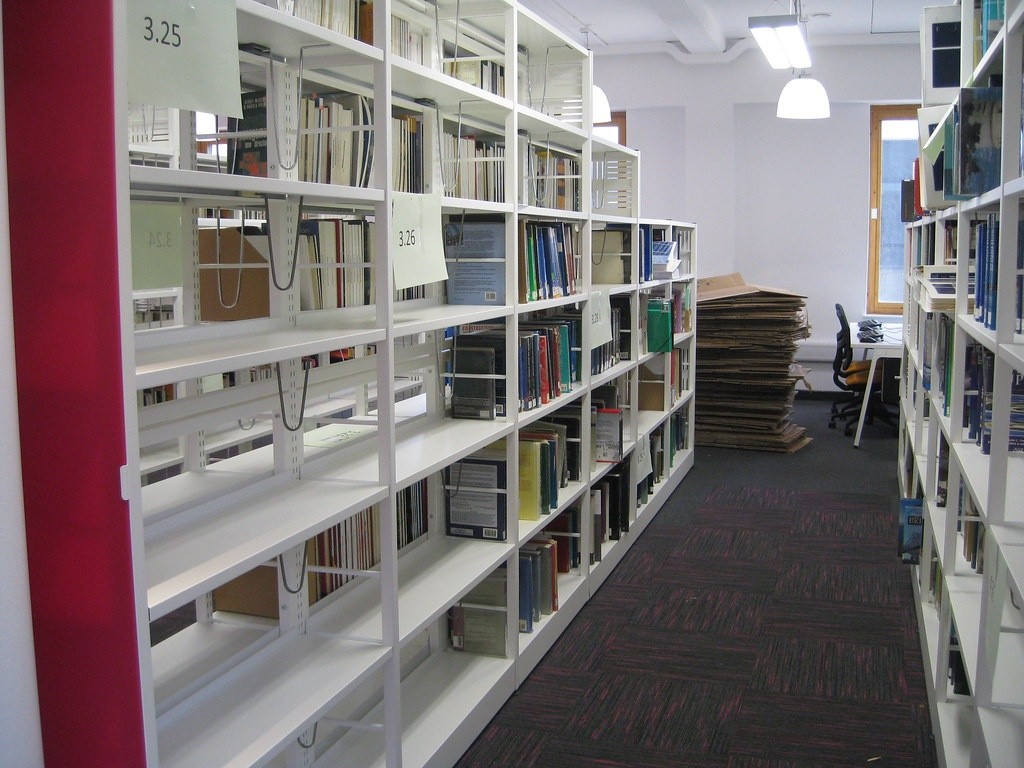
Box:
[892,2,1024,768]
[1,1,704,768]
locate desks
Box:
[850,313,905,448]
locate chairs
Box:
[827,302,901,437]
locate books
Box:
[898,1,1024,695]
[125,0,688,660]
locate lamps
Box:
[775,2,832,123]
[747,0,814,72]
